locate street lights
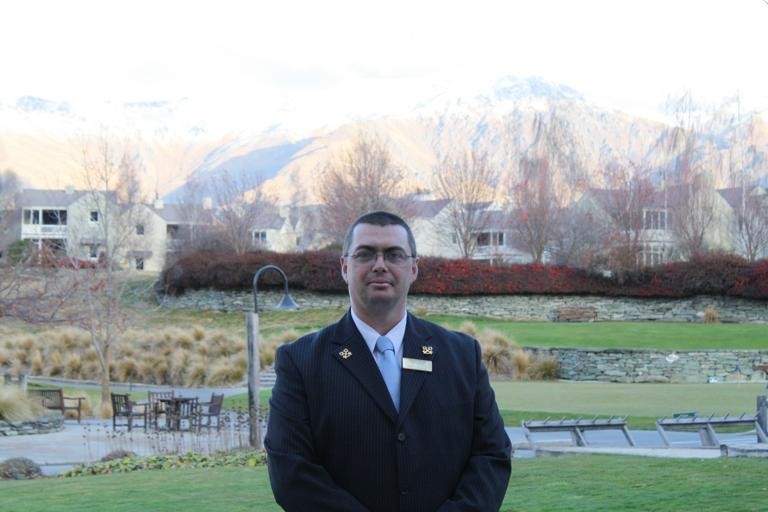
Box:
[246,264,299,451]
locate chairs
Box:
[107,389,227,435]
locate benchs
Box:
[27,387,85,425]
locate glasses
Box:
[343,248,413,265]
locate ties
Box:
[376,336,402,415]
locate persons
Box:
[263,211,512,511]
